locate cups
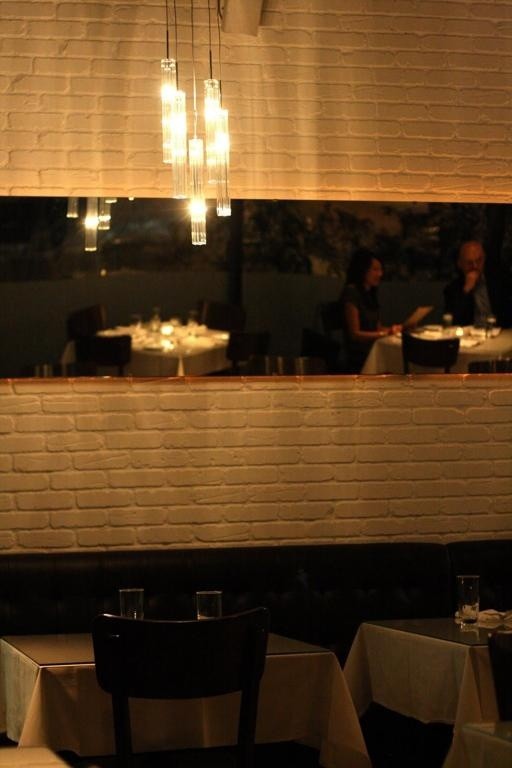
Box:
[119,589,145,623]
[457,574,480,629]
[195,590,222,622]
[441,310,497,346]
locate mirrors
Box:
[0,539,511,668]
[0,196,512,373]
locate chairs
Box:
[197,301,248,353]
[317,299,360,374]
[400,329,460,373]
[64,303,110,357]
[91,607,270,767]
[485,628,512,722]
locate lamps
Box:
[65,197,136,252]
[157,0,233,248]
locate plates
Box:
[116,307,204,353]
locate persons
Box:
[443,240,512,330]
[341,253,403,366]
